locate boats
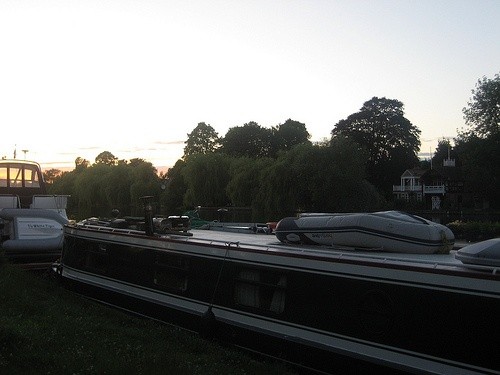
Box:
[274,209,457,254]
[0,143,76,237]
